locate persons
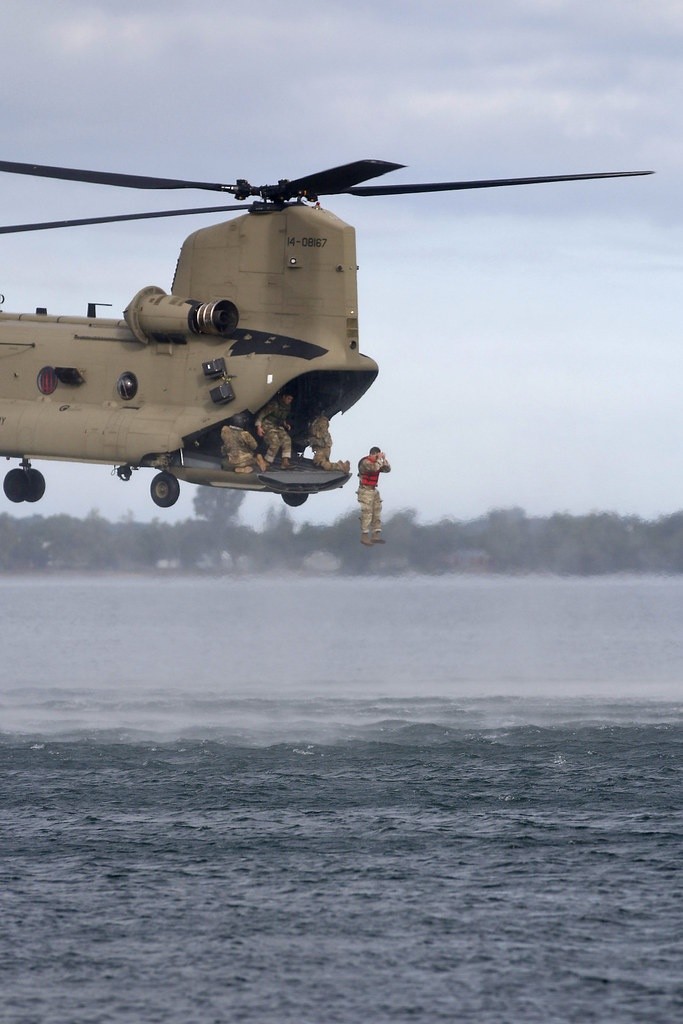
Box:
[306,400,350,474]
[356,446,392,546]
[254,389,298,469]
[221,412,259,474]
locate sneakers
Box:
[342,460,350,477]
[233,465,254,474]
[257,454,266,472]
[280,459,297,470]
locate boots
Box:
[360,532,386,546]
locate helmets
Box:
[229,413,250,430]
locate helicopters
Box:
[0,160,655,507]
